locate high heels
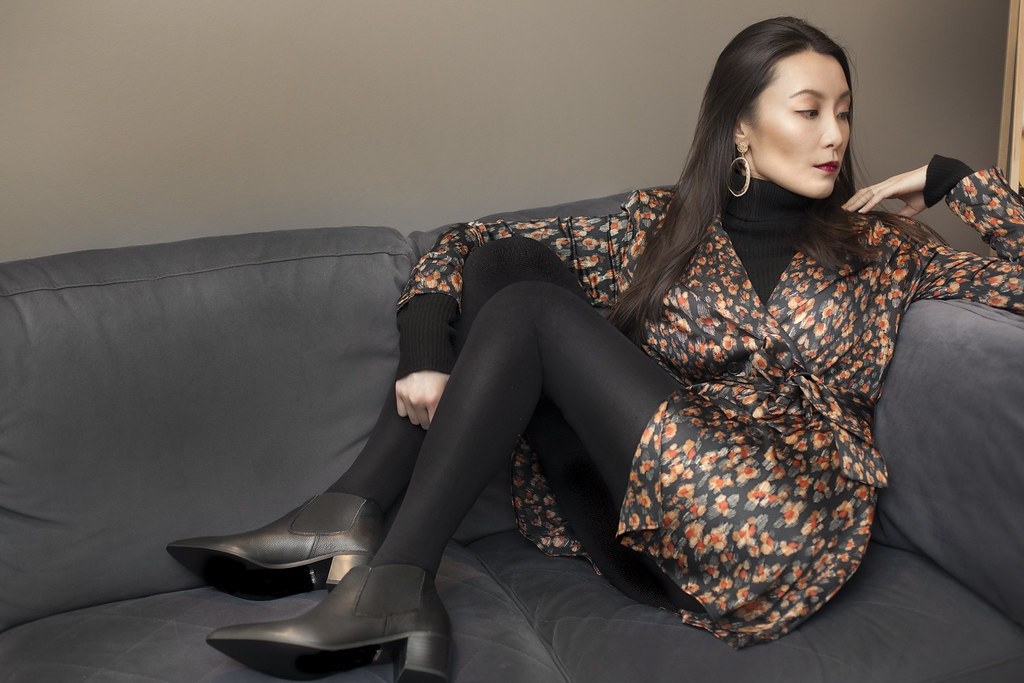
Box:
[165,493,383,601]
[205,564,453,681]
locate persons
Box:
[165,16,1023,683]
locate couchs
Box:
[0,179,1022,683]
